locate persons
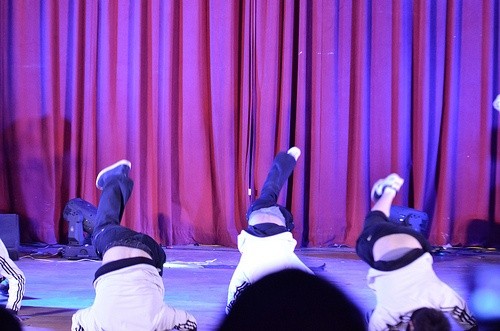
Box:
[404,308,454,331]
[218,147,366,331]
[69,160,198,331]
[213,267,370,330]
[354,174,478,331]
[0,239,26,331]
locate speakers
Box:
[0,214,20,248]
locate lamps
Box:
[62,198,98,246]
